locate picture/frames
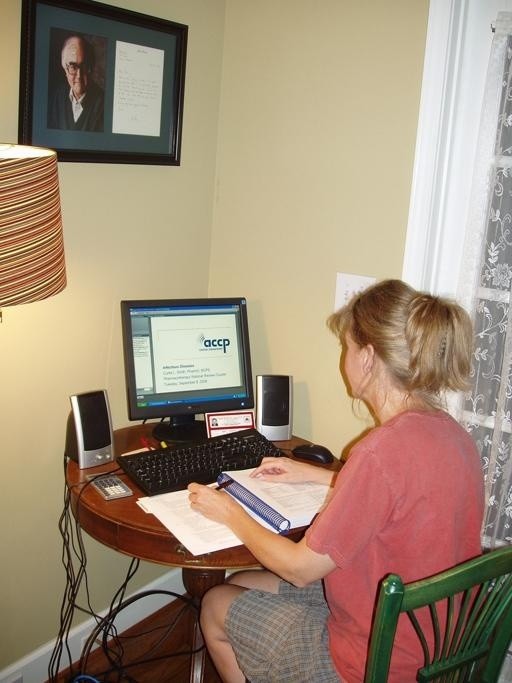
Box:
[16,0,190,166]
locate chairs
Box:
[362,541,512,682]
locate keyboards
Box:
[116,429,289,497]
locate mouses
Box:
[292,445,334,464]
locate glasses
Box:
[63,61,91,75]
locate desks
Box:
[66,419,345,683]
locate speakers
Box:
[256,374,293,442]
[65,390,114,469]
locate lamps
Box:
[0,143,68,325]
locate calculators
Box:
[85,471,134,501]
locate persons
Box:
[188,279,488,683]
[47,35,105,133]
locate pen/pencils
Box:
[215,479,235,491]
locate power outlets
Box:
[334,273,376,318]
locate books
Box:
[137,465,335,559]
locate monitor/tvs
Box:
[121,298,254,444]
